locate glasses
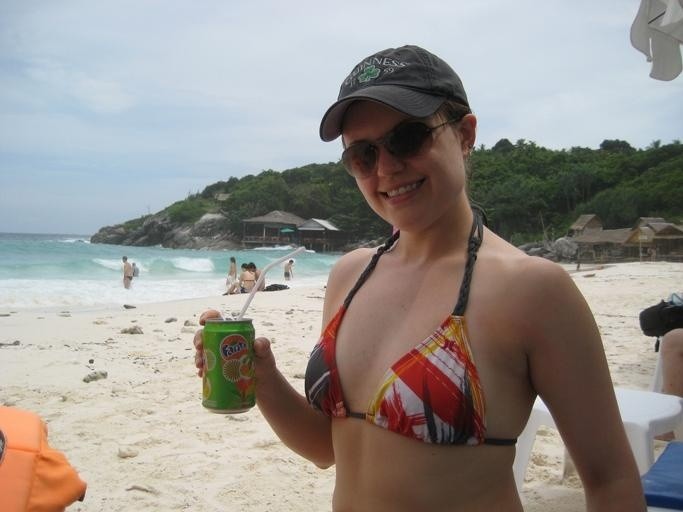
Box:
[341,120,457,178]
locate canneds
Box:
[202,318,256,414]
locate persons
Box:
[653,325,682,442]
[283,259,293,280]
[121,256,133,290]
[247,263,265,292]
[131,263,138,278]
[221,263,256,296]
[226,257,236,289]
[191,42,649,512]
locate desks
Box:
[512,388,683,490]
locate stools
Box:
[642,442,683,512]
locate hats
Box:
[317,44,468,141]
